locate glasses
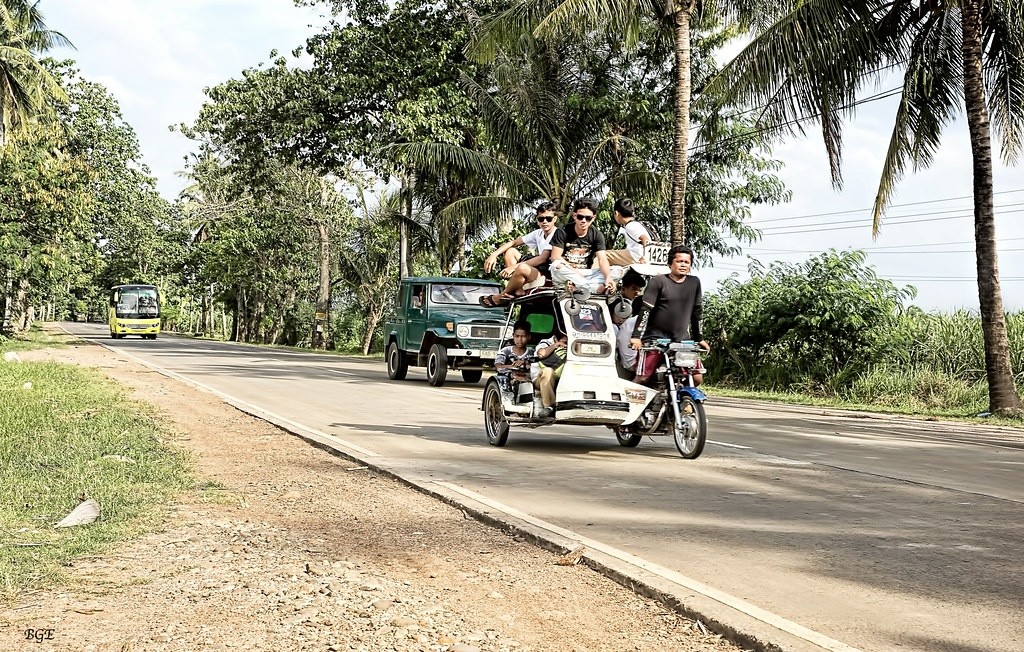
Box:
[537,216,554,222]
[576,213,594,222]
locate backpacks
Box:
[538,342,568,379]
[625,220,662,245]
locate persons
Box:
[479,203,565,308]
[549,199,616,294]
[530,328,567,416]
[606,268,650,372]
[413,291,423,308]
[591,198,652,268]
[494,321,535,405]
[630,245,710,388]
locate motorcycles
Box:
[480,282,713,459]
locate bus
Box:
[108,285,162,340]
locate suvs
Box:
[383,277,517,385]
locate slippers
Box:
[478,295,511,308]
[513,293,526,298]
[538,406,556,418]
